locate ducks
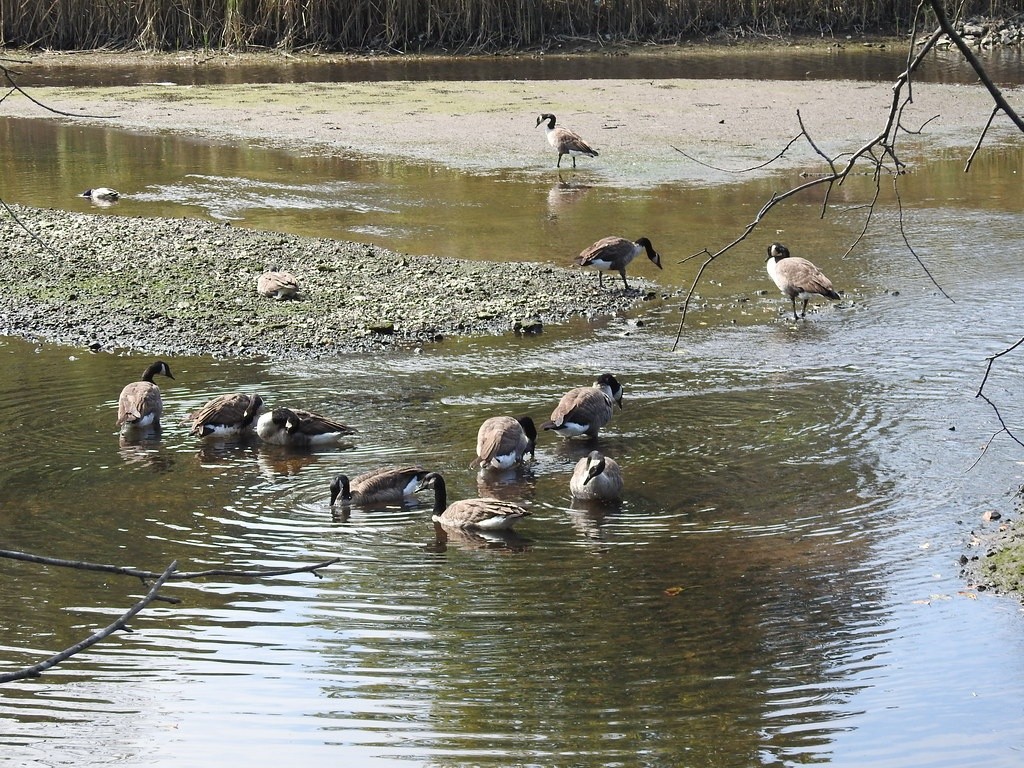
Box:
[188,393,265,438]
[329,465,434,507]
[540,374,625,445]
[570,450,625,502]
[468,417,537,472]
[535,114,599,167]
[764,242,841,321]
[256,407,358,448]
[114,361,176,429]
[415,472,533,531]
[256,265,301,300]
[82,188,121,201]
[573,236,663,288]
